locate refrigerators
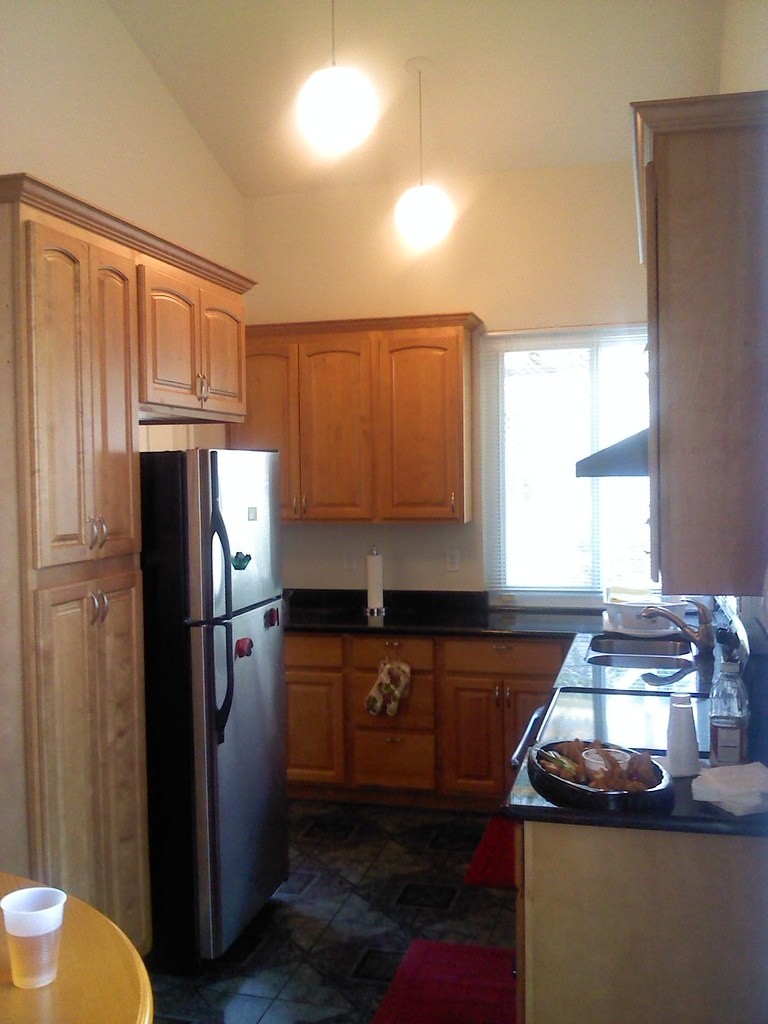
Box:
[139,450,290,963]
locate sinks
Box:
[582,634,695,670]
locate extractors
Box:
[576,427,649,476]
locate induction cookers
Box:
[535,653,768,758]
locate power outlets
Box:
[446,551,458,571]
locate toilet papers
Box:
[365,553,384,609]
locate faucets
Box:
[641,599,715,654]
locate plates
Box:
[529,738,673,801]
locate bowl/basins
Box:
[582,748,632,772]
[603,601,688,633]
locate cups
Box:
[665,691,701,779]
[0,886,68,990]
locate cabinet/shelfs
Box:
[629,90,768,596]
[228,318,374,526]
[140,222,256,420]
[0,172,141,568]
[0,570,148,960]
[376,311,483,524]
[282,629,768,1024]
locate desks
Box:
[0,872,154,1024]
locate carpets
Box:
[467,808,517,892]
[370,936,516,1024]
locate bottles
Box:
[708,662,752,768]
